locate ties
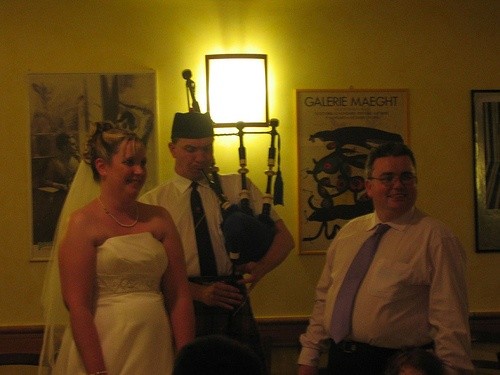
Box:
[330,223,391,345]
[189,182,218,284]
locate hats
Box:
[171,112,213,138]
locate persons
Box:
[394,348,446,375]
[131,108,296,366]
[171,330,268,374]
[299,143,478,375]
[57,119,198,375]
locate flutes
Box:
[180,69,284,297]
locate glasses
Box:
[367,173,417,185]
